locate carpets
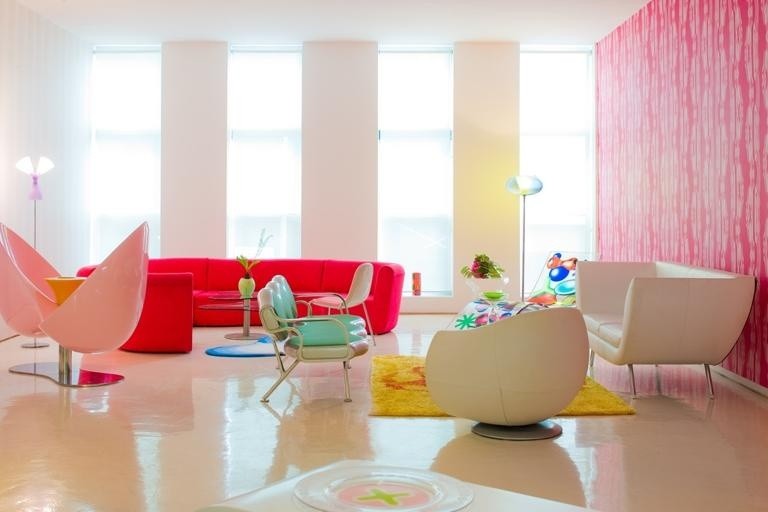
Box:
[368,355,638,417]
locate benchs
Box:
[257,274,369,403]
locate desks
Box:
[198,294,259,341]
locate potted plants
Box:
[236,254,261,296]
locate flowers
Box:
[459,253,506,279]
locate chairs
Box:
[426,307,590,441]
[1,220,150,389]
[306,263,376,347]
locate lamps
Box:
[505,175,543,303]
[14,155,56,250]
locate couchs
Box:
[76,257,405,354]
[575,259,756,399]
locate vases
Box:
[471,271,489,278]
